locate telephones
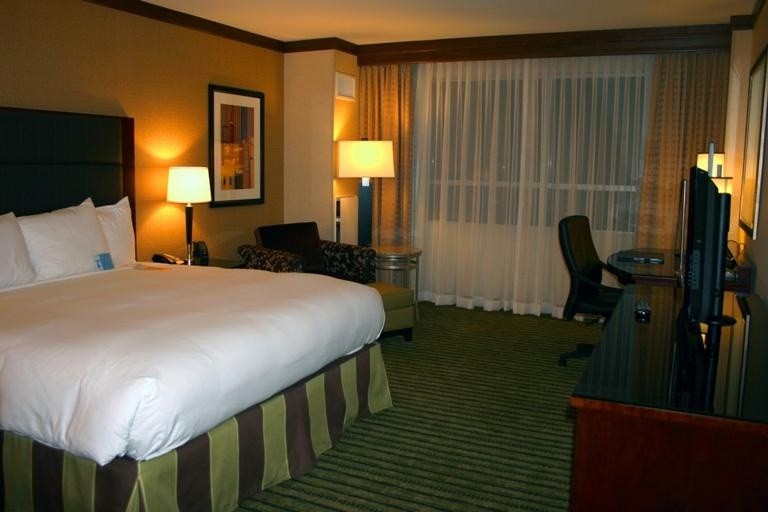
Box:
[152,253,187,265]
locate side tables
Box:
[375,246,423,322]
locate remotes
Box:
[635,299,651,316]
[634,310,651,323]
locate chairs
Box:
[557,214,626,330]
[237,221,412,346]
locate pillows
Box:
[0,193,139,294]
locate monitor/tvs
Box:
[663,310,722,414]
[679,165,736,326]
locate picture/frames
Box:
[206,83,265,208]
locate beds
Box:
[0,104,388,511]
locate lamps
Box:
[333,138,397,248]
[694,152,726,194]
[167,166,212,266]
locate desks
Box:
[568,247,766,510]
